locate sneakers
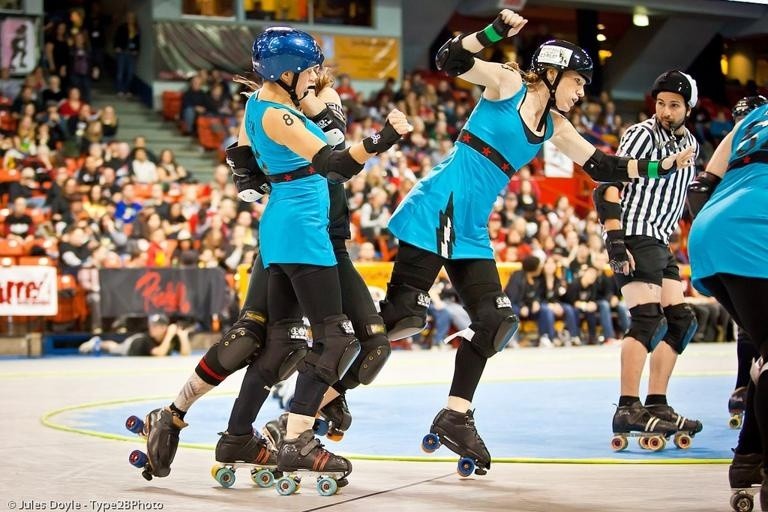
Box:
[505,340,520,348]
[538,334,556,348]
[78,335,101,355]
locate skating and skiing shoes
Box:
[422,406,491,477]
[260,412,291,450]
[211,428,278,488]
[610,400,678,452]
[729,447,768,511]
[728,387,748,429]
[759,467,768,511]
[277,430,352,495]
[126,407,188,480]
[286,388,352,441]
[639,403,703,449]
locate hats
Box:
[149,313,171,325]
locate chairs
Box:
[162,91,184,118]
[422,302,628,345]
[0,153,87,335]
[197,116,225,148]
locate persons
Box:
[1,7,768,511]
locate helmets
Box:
[650,71,699,109]
[250,24,325,82]
[531,40,595,86]
[731,94,768,116]
